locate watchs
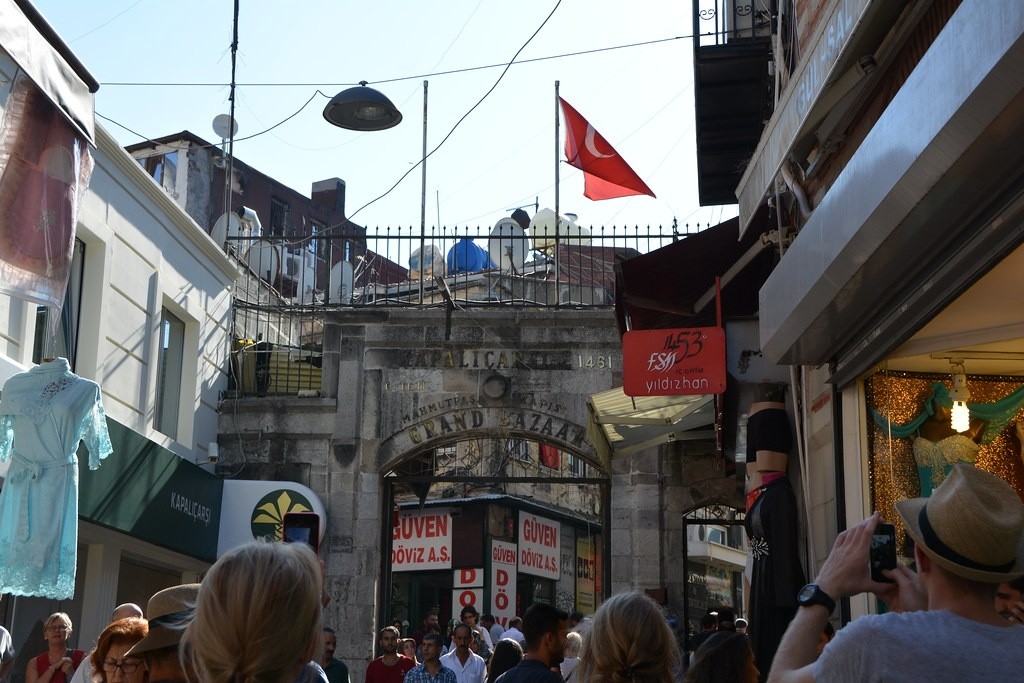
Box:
[799,585,835,615]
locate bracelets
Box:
[49,666,55,672]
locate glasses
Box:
[98,656,143,674]
[48,625,70,632]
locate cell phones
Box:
[869,523,897,585]
[283,513,320,560]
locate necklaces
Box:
[48,655,62,663]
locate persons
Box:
[684,608,761,683]
[366,606,528,683]
[26,612,87,683]
[0,625,16,683]
[577,592,676,683]
[766,466,1024,683]
[495,603,569,683]
[745,451,804,683]
[317,627,349,683]
[69,583,198,683]
[179,541,322,683]
[559,610,584,683]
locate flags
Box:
[557,96,657,201]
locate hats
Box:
[895,463,1024,583]
[124,583,202,659]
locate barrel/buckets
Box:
[488,218,529,271]
[531,208,590,253]
[410,245,446,278]
[446,239,493,273]
